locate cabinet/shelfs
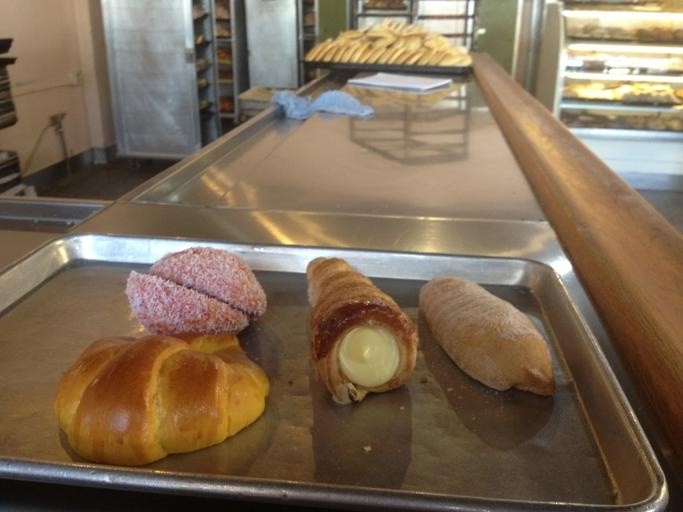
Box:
[100,0,320,161]
[534,1,683,193]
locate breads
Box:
[618,82,682,107]
[303,18,473,67]
[419,278,555,398]
[52,331,272,468]
[125,249,268,337]
[306,257,419,405]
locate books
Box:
[346,71,453,93]
